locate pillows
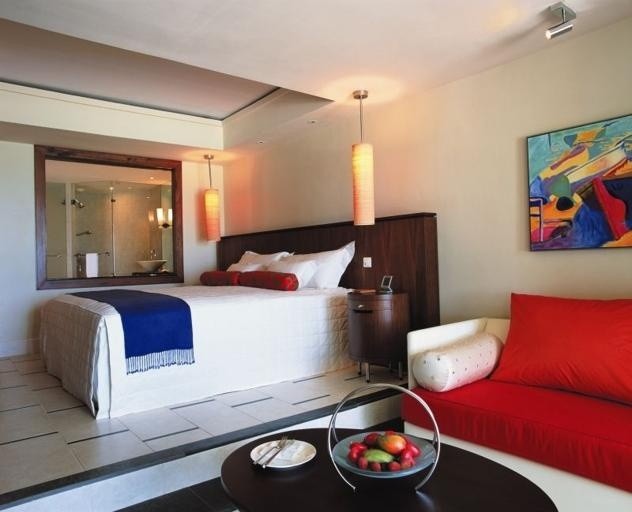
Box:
[200,241,355,291]
[413,331,503,393]
[488,292,631,404]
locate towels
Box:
[86,253,98,278]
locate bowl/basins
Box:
[332,430,435,479]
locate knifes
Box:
[262,439,295,468]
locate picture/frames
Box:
[526,113,632,251]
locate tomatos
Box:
[349,431,420,472]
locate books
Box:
[354,287,376,294]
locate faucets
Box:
[151,248,156,260]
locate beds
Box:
[39,212,441,420]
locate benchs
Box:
[400,316,632,512]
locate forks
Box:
[253,434,288,465]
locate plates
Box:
[249,438,318,469]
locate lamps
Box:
[546,2,576,39]
[204,154,221,243]
[156,207,173,229]
[350,89,375,226]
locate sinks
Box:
[135,260,167,272]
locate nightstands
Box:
[347,291,410,383]
